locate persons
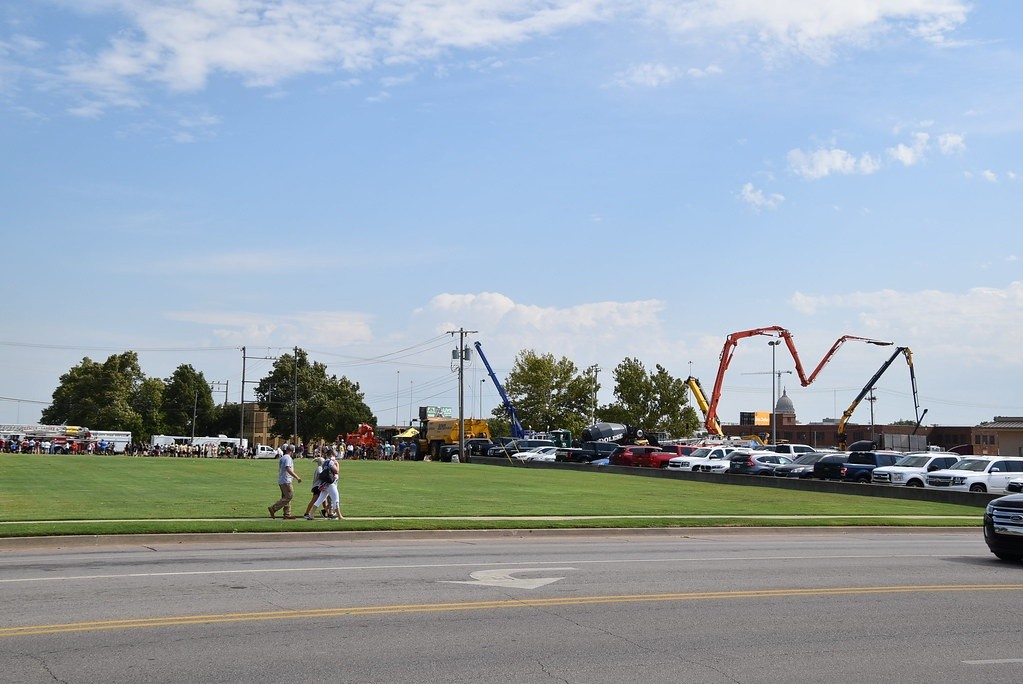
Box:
[0,438,417,462]
[267,445,301,520]
[304,448,349,520]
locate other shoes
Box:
[319,509,326,517]
[268,507,275,519]
[328,514,337,519]
[304,514,310,518]
[283,515,296,519]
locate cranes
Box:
[703,326,894,440]
[473,341,556,441]
[837,347,929,452]
[683,375,771,446]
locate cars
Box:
[983,480,1023,562]
[462,421,847,479]
[1003,479,1023,496]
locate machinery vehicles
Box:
[346,422,379,448]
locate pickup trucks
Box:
[813,450,907,485]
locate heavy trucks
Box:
[408,416,491,462]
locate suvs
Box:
[923,455,1023,494]
[870,450,961,489]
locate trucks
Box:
[248,443,280,460]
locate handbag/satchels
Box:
[319,459,335,483]
